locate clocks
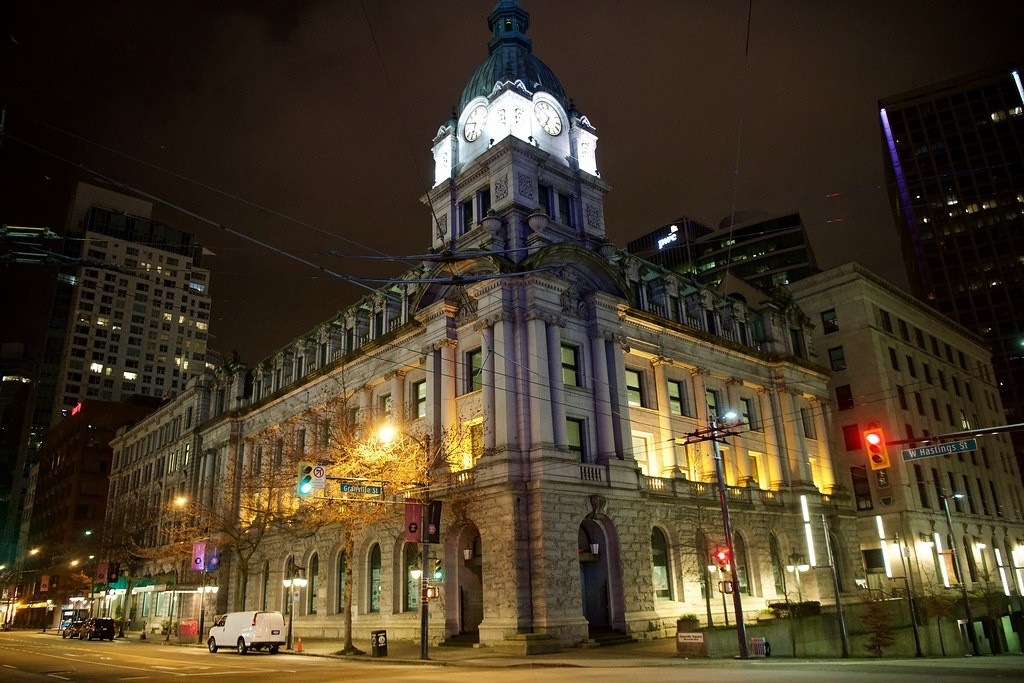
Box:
[462,105,489,143]
[534,101,563,137]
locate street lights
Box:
[933,492,1024,657]
[800,495,850,658]
[178,497,218,643]
[380,425,430,657]
[708,410,748,657]
[877,515,925,659]
[282,555,307,651]
[786,547,809,602]
[709,545,731,624]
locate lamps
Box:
[589,523,599,556]
[463,528,473,569]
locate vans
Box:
[208,611,286,655]
[79,616,114,640]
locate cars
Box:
[61,619,83,638]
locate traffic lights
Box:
[434,558,443,581]
[426,586,439,598]
[863,428,891,470]
[721,580,733,593]
[715,550,727,572]
[298,461,314,498]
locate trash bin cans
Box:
[371,630,387,657]
[748,637,765,658]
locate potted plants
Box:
[161,619,178,636]
[756,608,776,623]
[675,612,699,635]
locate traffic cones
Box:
[296,638,305,652]
